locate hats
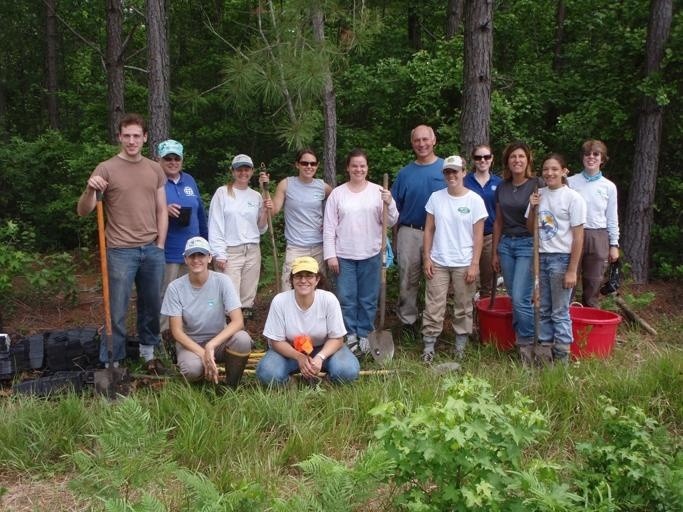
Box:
[158,140,183,160]
[232,154,253,169]
[182,236,211,257]
[290,256,319,274]
[443,156,462,170]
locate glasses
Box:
[299,161,317,166]
[444,170,458,176]
[583,152,600,156]
[293,274,315,279]
[473,154,492,160]
[163,157,180,161]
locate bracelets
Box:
[316,352,327,362]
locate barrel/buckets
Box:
[568,307,621,364]
[474,296,516,353]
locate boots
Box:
[216,348,251,397]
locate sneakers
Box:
[420,352,434,366]
[144,359,170,375]
[400,326,416,346]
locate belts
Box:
[405,225,425,231]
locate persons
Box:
[259,149,332,292]
[208,154,273,326]
[255,257,360,388]
[157,139,208,336]
[567,140,619,308]
[492,142,536,348]
[463,142,501,298]
[524,154,586,363]
[78,114,168,373]
[322,150,399,353]
[160,236,251,389]
[391,125,448,326]
[421,155,489,362]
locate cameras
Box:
[600,278,620,295]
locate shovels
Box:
[217,361,461,378]
[92,189,129,399]
[520,184,553,370]
[366,174,394,363]
